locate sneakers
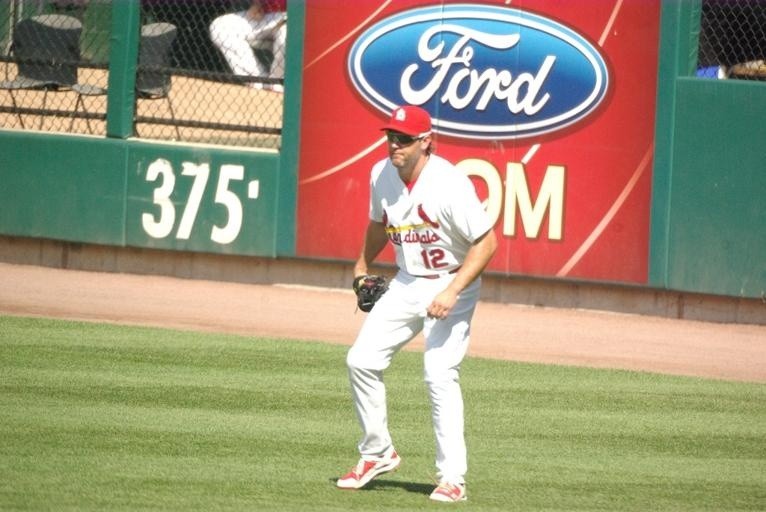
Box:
[429,481,468,502]
[336,444,401,490]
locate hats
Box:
[379,105,432,136]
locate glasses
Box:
[387,132,423,142]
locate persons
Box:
[209,1,288,91]
[334,106,498,505]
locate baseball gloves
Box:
[351,273,390,312]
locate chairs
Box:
[68,22,182,141]
[0,13,83,134]
